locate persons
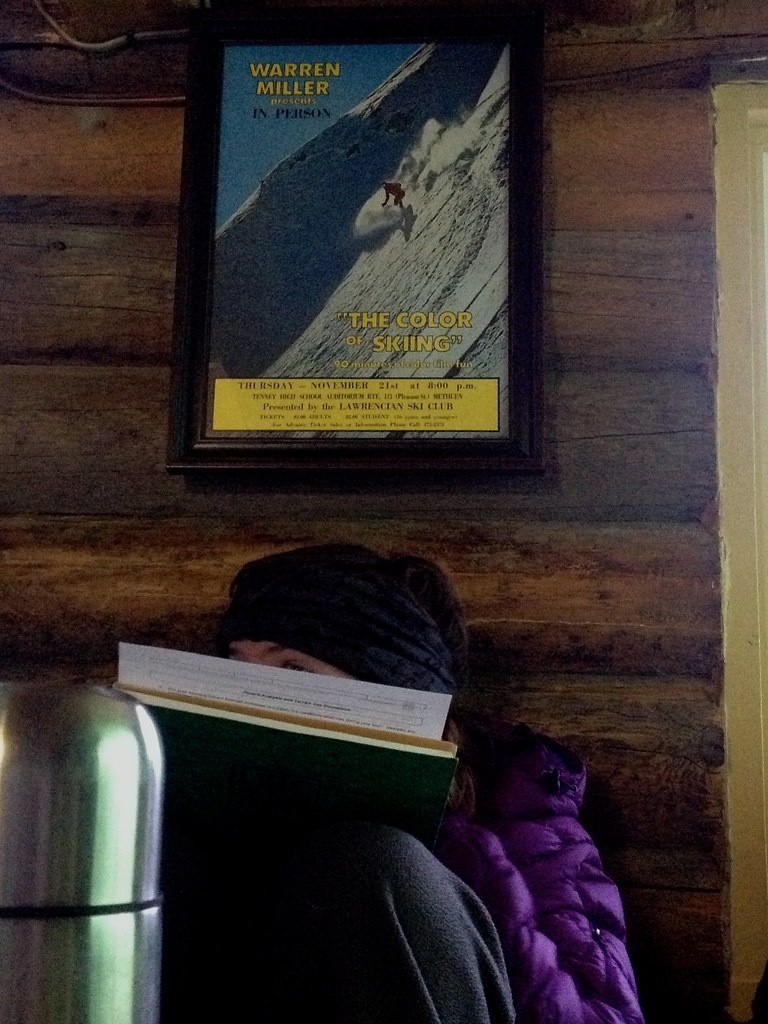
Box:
[221,541,642,1024]
[380,180,407,208]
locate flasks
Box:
[0,688,162,1024]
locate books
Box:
[111,640,460,854]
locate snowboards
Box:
[404,204,411,240]
